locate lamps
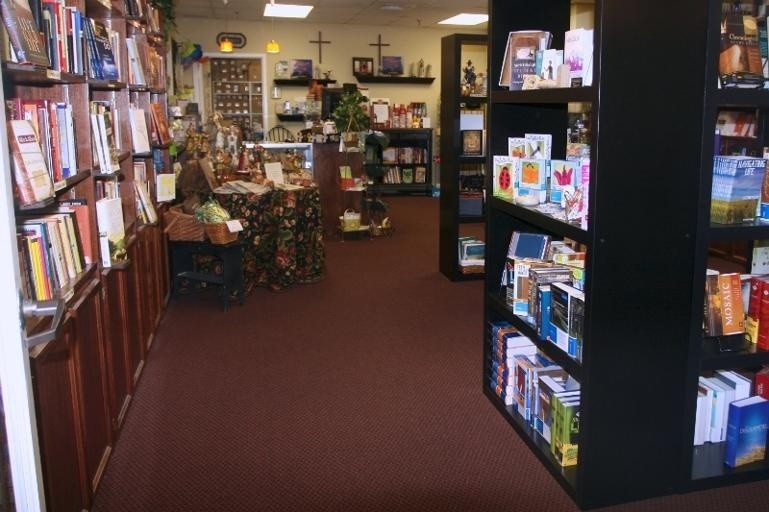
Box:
[265,0,280,53]
[219,0,233,52]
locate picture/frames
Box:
[352,56,374,76]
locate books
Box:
[380,144,429,185]
[198,156,305,196]
[1,0,183,319]
[486,1,593,469]
[458,98,485,274]
[692,0,767,482]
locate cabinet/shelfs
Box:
[274,73,436,122]
[372,129,432,197]
[439,33,488,280]
[483,0,707,512]
[687,0,769,487]
[0,0,171,512]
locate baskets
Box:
[163,203,208,242]
[204,219,239,245]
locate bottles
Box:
[388,102,427,128]
